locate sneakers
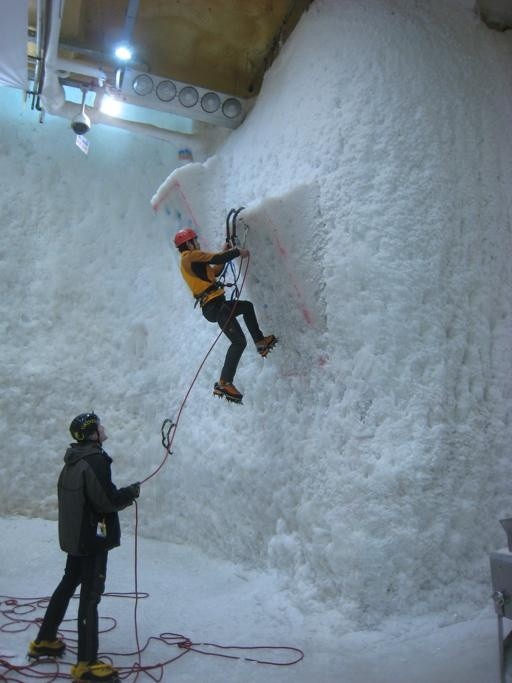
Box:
[214,379,243,398]
[255,335,275,352]
[28,638,67,657]
[74,660,119,682]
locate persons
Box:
[174,226,278,403]
[27,412,142,682]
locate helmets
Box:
[69,412,99,443]
[174,229,197,247]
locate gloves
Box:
[128,481,140,498]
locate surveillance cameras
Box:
[71,113,91,134]
